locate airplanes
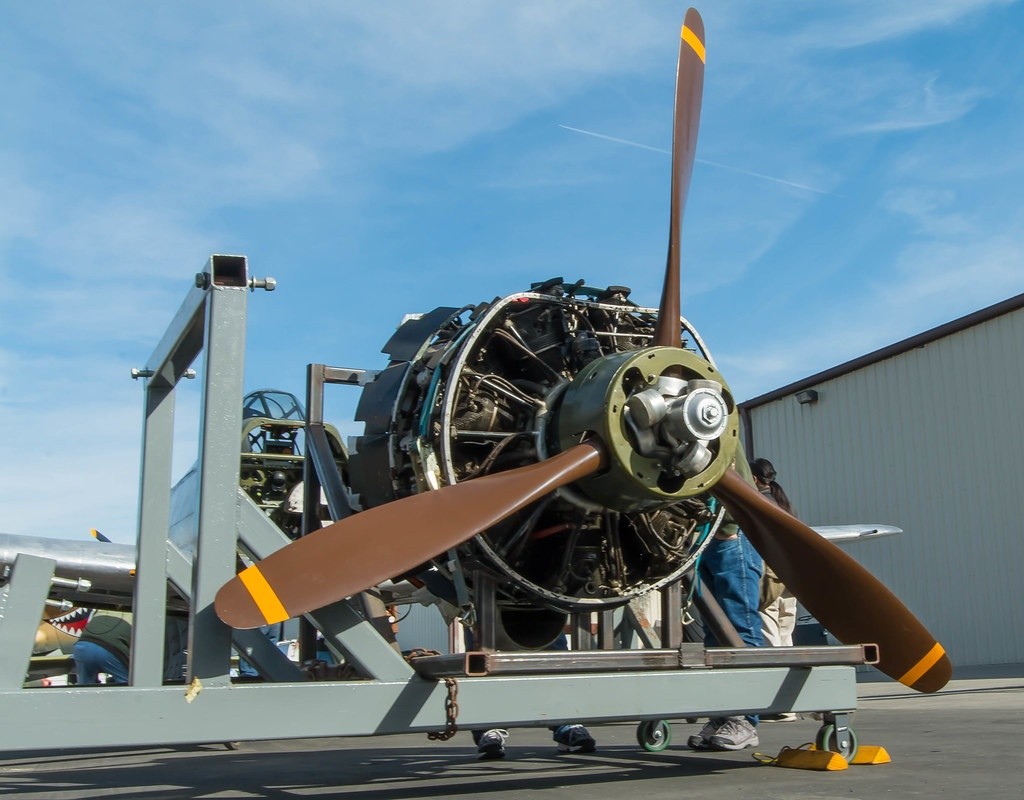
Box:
[0,6,954,695]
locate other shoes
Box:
[760,712,797,723]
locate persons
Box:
[72,608,133,686]
[239,622,276,677]
[687,439,764,750]
[41,674,51,686]
[749,458,797,723]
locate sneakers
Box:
[709,715,759,750]
[477,729,509,759]
[557,724,595,751]
[688,719,725,750]
